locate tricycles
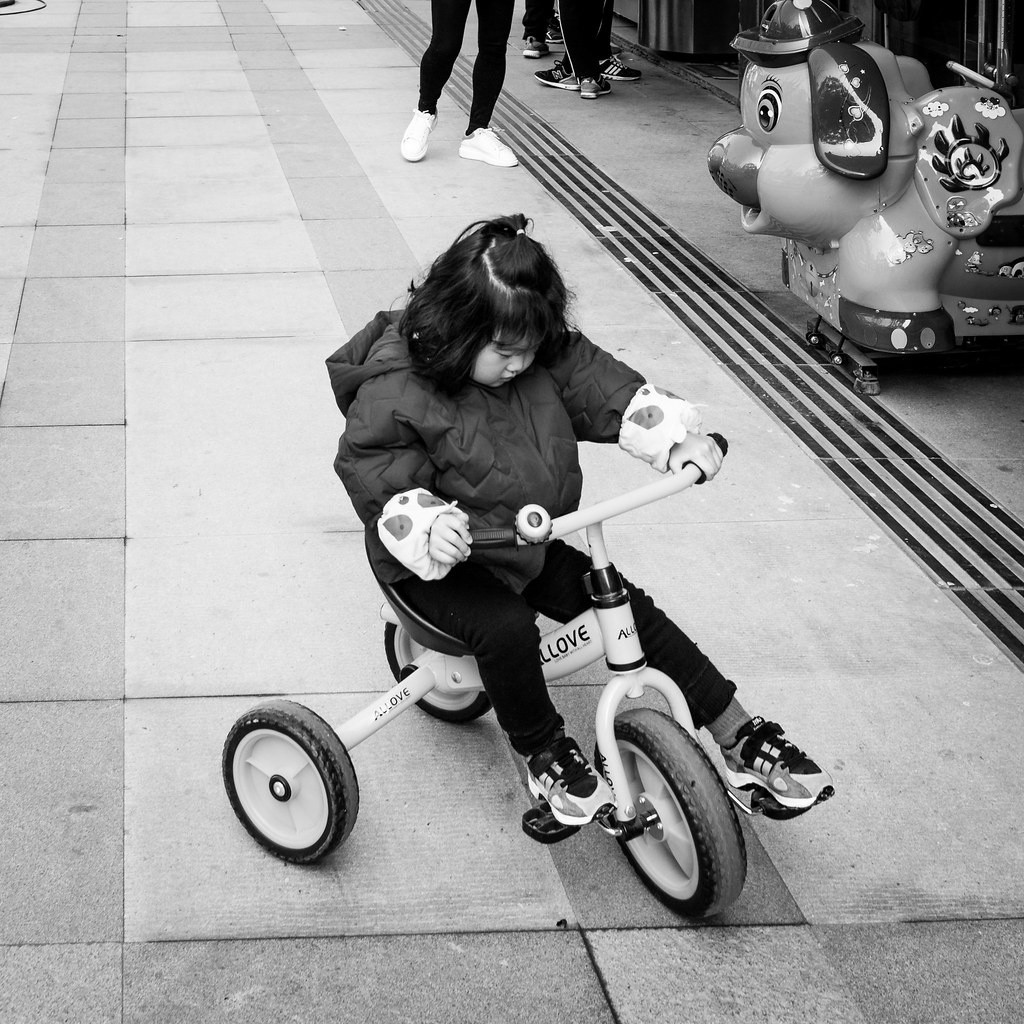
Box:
[223,434,748,920]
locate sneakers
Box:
[401,107,439,162]
[600,54,642,80]
[546,16,564,44]
[526,737,619,826]
[459,128,519,167]
[523,36,549,58]
[533,60,581,90]
[580,74,612,98]
[720,716,835,808]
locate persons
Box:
[399,1,519,169]
[534,0,611,99]
[521,0,564,59]
[323,212,832,834]
[599,0,643,81]
